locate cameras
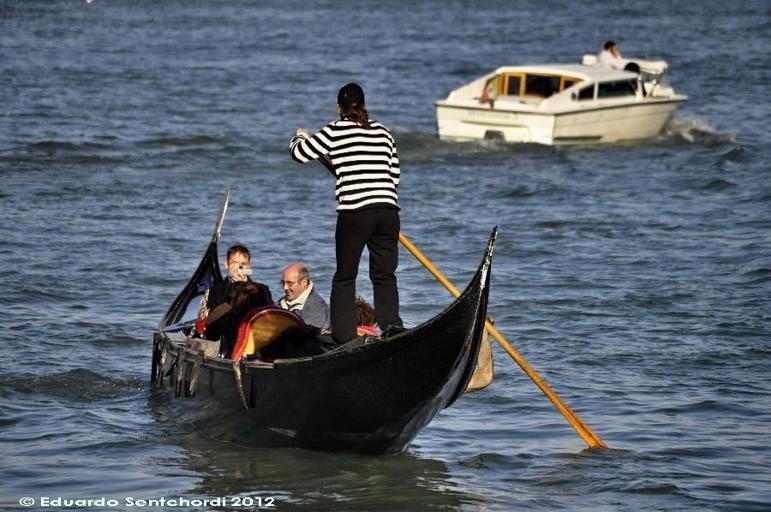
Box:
[237,265,252,276]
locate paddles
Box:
[317,156,607,453]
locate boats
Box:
[433,55,688,145]
[151,187,499,456]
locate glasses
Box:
[280,276,306,287]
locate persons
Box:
[598,40,625,70]
[289,82,403,343]
[354,293,379,336]
[221,281,268,362]
[207,281,246,323]
[204,245,276,316]
[615,63,648,97]
[273,262,332,331]
